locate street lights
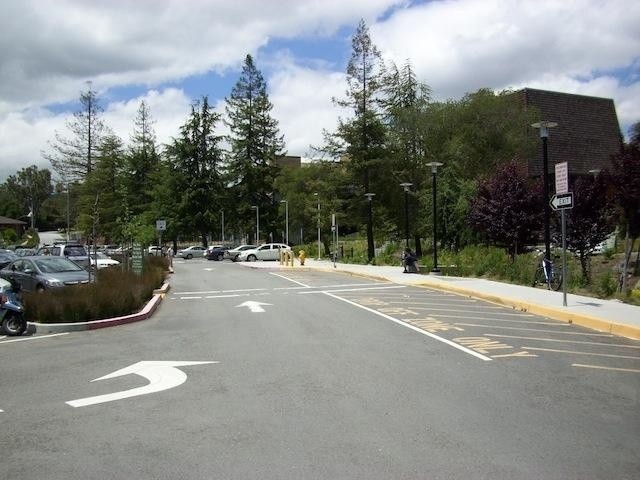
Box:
[280,199,289,246]
[400,182,413,247]
[251,204,259,246]
[424,161,443,273]
[220,207,226,244]
[532,120,557,282]
[365,193,376,265]
[314,191,321,259]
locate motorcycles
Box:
[0,265,27,336]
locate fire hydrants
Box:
[299,250,305,266]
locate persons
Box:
[403,246,420,272]
[167,245,174,267]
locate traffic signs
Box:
[549,192,574,211]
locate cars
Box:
[175,243,292,262]
[0,242,168,296]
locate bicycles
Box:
[531,248,563,291]
